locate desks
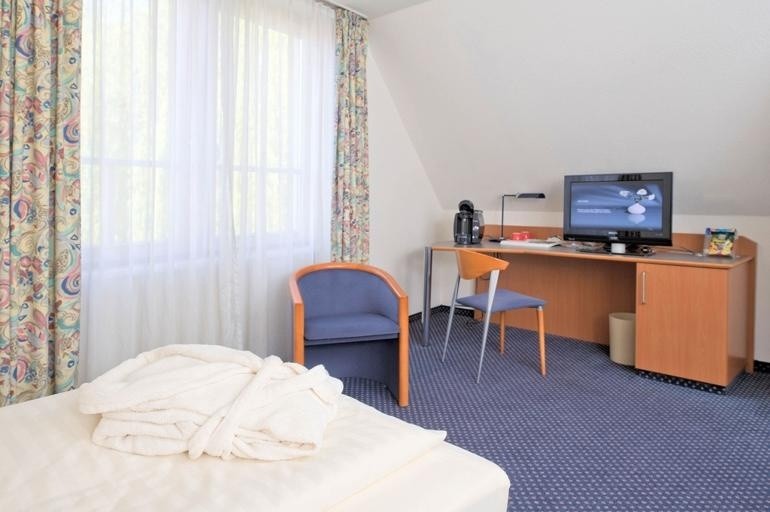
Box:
[421,225,758,395]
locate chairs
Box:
[288,262,409,408]
[442,248,547,384]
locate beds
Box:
[0,345,510,512]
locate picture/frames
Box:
[703,227,738,258]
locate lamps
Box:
[489,193,545,243]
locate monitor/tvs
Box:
[563,172,673,255]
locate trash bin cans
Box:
[609,312,635,366]
